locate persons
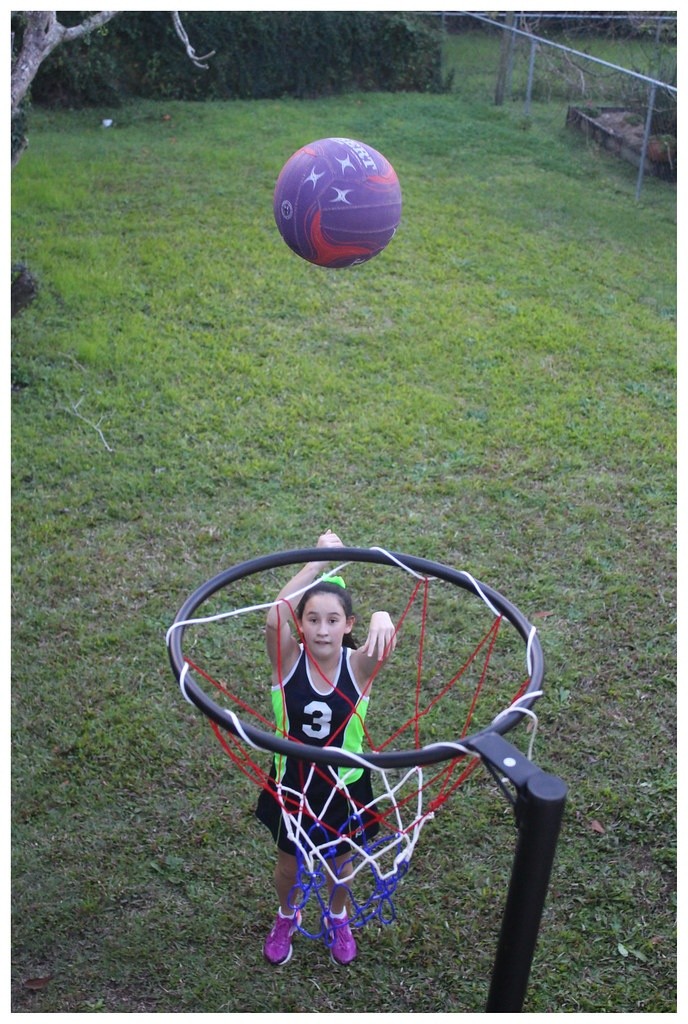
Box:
[255,528,397,965]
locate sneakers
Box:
[323,912,356,965]
[262,904,303,965]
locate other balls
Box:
[270,135,403,271]
